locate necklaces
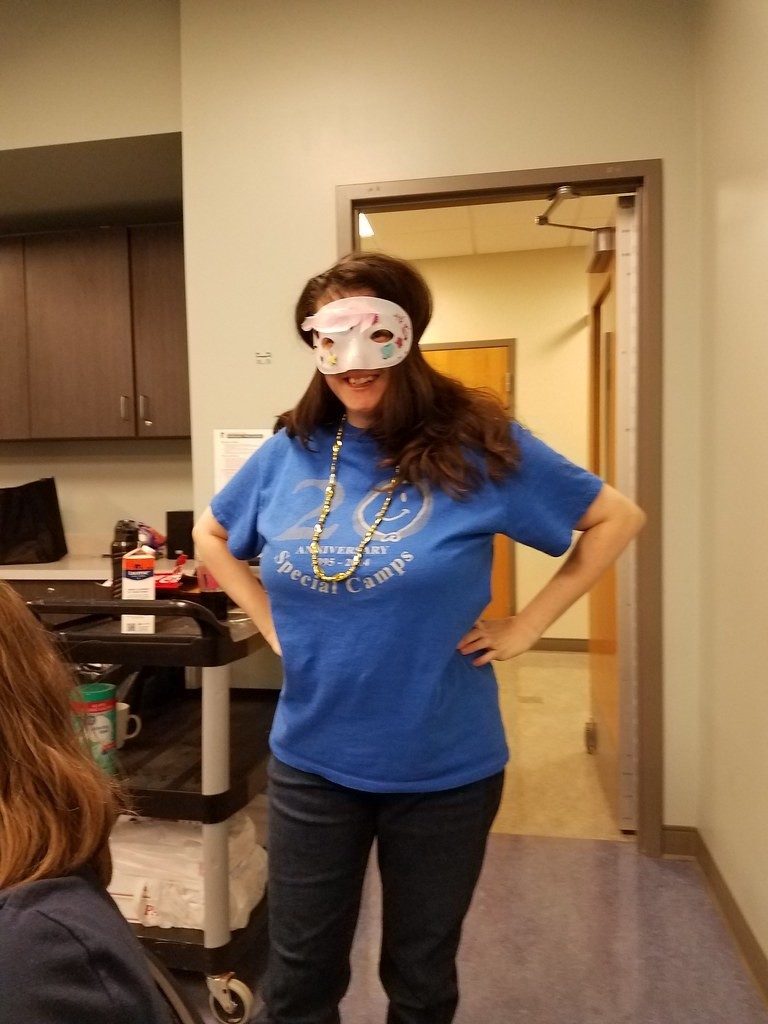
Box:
[310,413,404,582]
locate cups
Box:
[198,589,227,638]
[116,702,142,750]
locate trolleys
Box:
[24,590,282,1024]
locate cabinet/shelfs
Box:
[24,204,191,441]
[0,223,24,441]
[21,591,286,1024]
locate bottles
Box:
[109,520,139,620]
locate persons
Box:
[0,582,179,1023]
[190,256,647,1024]
